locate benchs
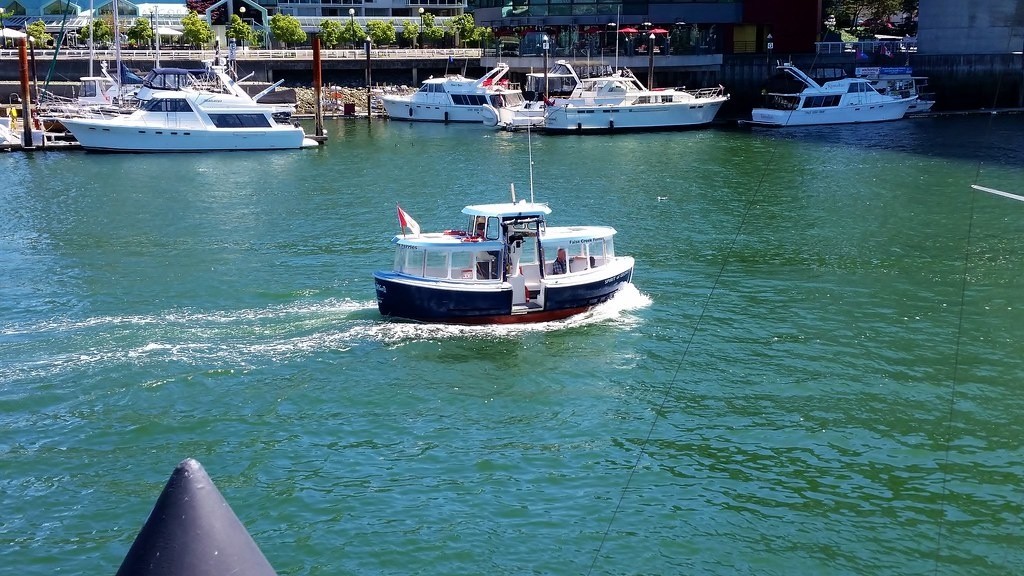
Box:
[524,282,540,302]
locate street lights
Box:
[150,7,154,50]
[349,8,356,50]
[240,6,246,55]
[418,7,425,47]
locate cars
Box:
[497,41,519,57]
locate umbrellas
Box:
[647,28,669,34]
[618,28,638,33]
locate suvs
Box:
[519,31,553,57]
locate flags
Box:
[396,205,421,238]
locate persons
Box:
[622,33,640,56]
[583,256,598,271]
[553,249,579,275]
[660,33,673,57]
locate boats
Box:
[58,63,321,153]
[0,123,22,150]
[377,61,524,123]
[854,66,935,115]
[738,56,918,127]
[495,60,640,130]
[538,33,733,137]
[372,112,636,330]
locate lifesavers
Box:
[651,87,667,92]
[442,229,468,236]
[460,236,487,243]
